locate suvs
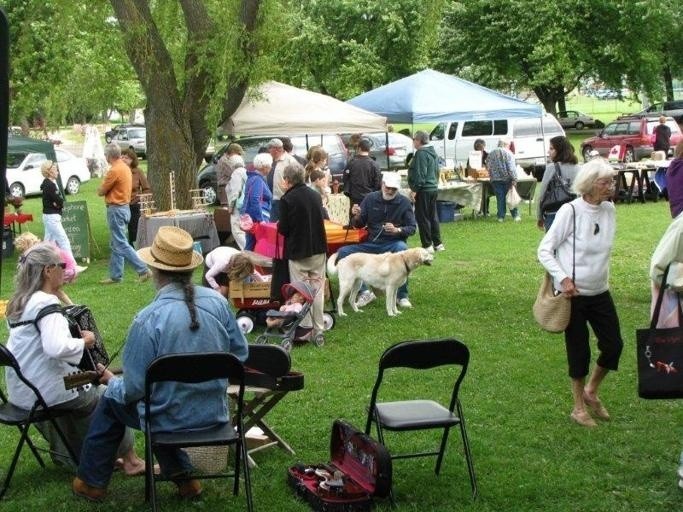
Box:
[580,115,683,163]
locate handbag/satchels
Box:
[636,263,683,399]
[239,213,254,231]
[541,161,577,212]
[532,271,572,332]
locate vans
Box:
[429,113,566,181]
[197,133,349,206]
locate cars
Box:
[554,110,594,130]
[105,124,144,143]
[111,128,146,156]
[599,90,618,99]
[340,133,417,169]
[644,100,682,122]
[6,149,91,197]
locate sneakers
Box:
[76,266,88,272]
[99,278,121,284]
[435,244,445,251]
[426,245,434,253]
[396,297,412,307]
[356,290,375,307]
[571,409,596,427]
[583,390,609,418]
[136,269,152,283]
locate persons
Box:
[409,131,446,253]
[4,243,160,476]
[41,159,87,276]
[97,142,154,284]
[665,141,682,218]
[69,226,248,503]
[653,116,670,159]
[537,158,623,427]
[276,163,329,344]
[465,138,522,223]
[263,291,307,329]
[649,210,683,488]
[536,136,583,233]
[201,133,416,306]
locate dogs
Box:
[326,247,434,317]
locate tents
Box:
[218,79,391,173]
[342,69,548,171]
[6,135,68,204]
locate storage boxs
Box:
[229,280,271,299]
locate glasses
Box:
[595,180,617,187]
[50,263,66,269]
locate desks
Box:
[321,221,370,314]
[449,173,537,222]
[401,181,486,222]
[613,166,669,204]
[132,210,220,254]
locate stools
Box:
[223,381,298,470]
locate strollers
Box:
[254,276,327,351]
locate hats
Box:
[228,143,245,155]
[267,138,283,147]
[136,226,204,273]
[382,173,401,189]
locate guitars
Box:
[64,366,123,393]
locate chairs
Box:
[365,339,478,511]
[0,340,81,497]
[144,354,254,511]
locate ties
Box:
[267,159,276,191]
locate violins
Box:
[313,463,367,500]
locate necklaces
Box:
[582,206,602,236]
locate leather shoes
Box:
[179,480,201,498]
[73,477,107,501]
